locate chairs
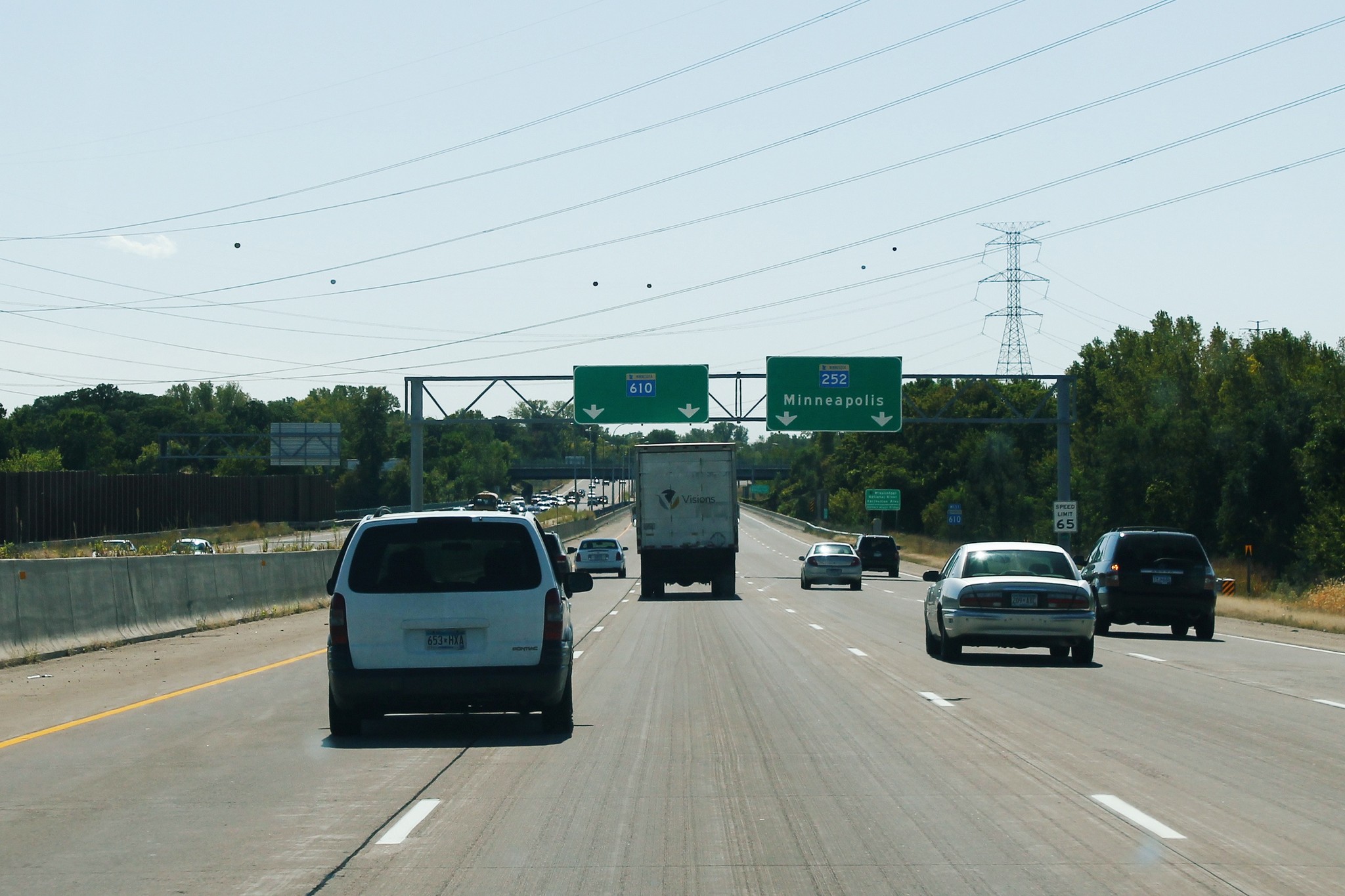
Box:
[1029,563,1051,575]
[384,550,427,594]
[482,540,539,591]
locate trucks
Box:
[630,441,741,598]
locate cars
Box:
[922,542,1098,665]
[798,541,862,590]
[92,539,138,557]
[544,530,576,574]
[171,537,216,554]
[574,538,628,579]
[452,478,626,515]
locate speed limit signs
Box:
[1052,500,1079,533]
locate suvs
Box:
[853,534,901,578]
[1071,524,1219,640]
[325,505,593,736]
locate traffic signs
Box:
[765,354,905,435]
[572,363,710,427]
